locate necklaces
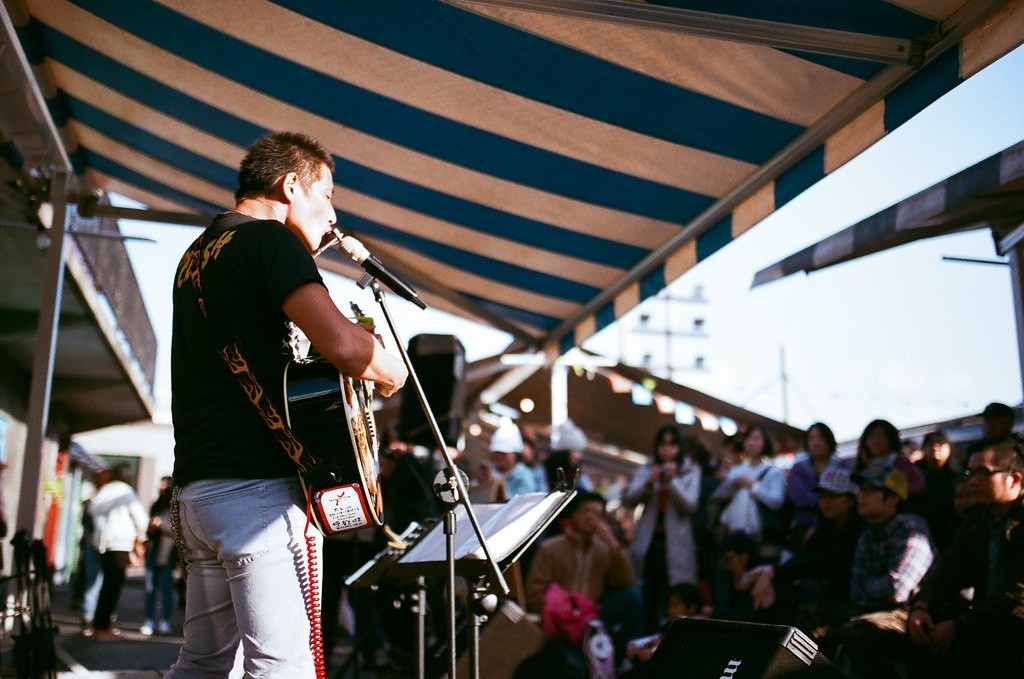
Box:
[236,195,277,222]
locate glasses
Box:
[853,484,885,496]
[815,490,842,499]
[656,439,679,448]
[959,467,1011,480]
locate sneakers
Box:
[92,628,129,640]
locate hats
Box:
[816,467,853,494]
[488,423,522,453]
[558,419,587,449]
[849,459,907,500]
[976,403,1015,421]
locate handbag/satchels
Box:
[717,487,763,536]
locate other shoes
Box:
[159,622,172,634]
[140,625,153,636]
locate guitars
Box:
[281,300,384,538]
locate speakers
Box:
[415,595,591,679]
[631,615,852,679]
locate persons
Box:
[72,468,117,638]
[159,129,412,679]
[139,476,176,636]
[318,399,1024,678]
[88,458,148,641]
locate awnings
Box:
[748,131,1024,288]
[0,0,1024,634]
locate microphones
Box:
[340,236,428,310]
